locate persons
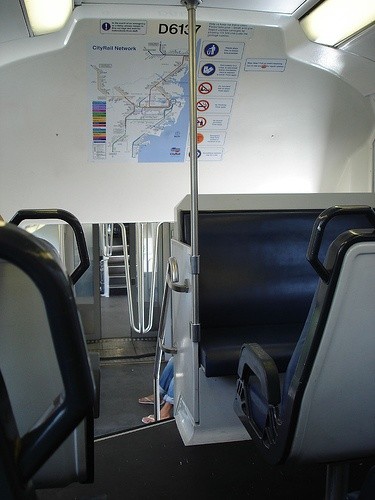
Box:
[137,354,178,424]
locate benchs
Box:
[181,210,375,378]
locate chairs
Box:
[231,203,374,500]
[0,208,105,500]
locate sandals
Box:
[138,396,165,405]
[142,415,169,423]
